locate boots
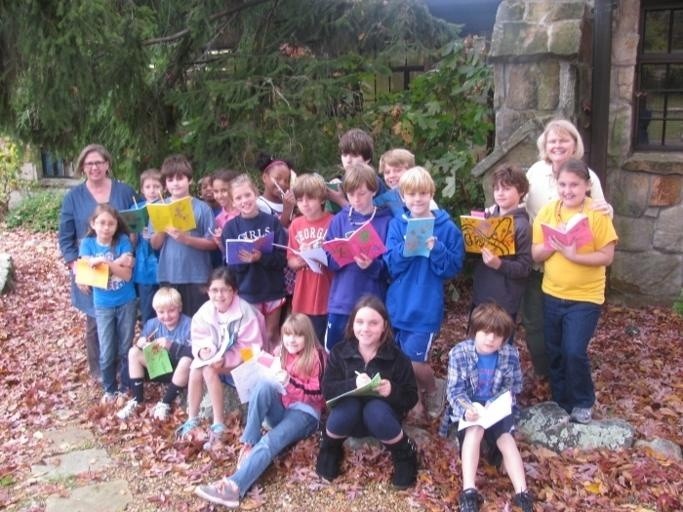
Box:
[382,431,417,490]
[315,428,347,481]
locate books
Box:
[460,215,516,256]
[326,372,382,410]
[190,328,234,370]
[326,182,347,214]
[540,213,593,253]
[75,257,109,291]
[271,241,328,275]
[402,217,435,258]
[231,350,287,404]
[118,199,161,233]
[323,221,387,269]
[208,227,225,254]
[146,196,196,231]
[225,231,274,266]
[457,391,513,432]
[142,339,173,379]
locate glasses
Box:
[84,160,107,166]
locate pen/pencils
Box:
[270,176,286,198]
[146,327,159,341]
[456,398,472,411]
[158,190,167,206]
[353,368,362,378]
[401,213,410,223]
[130,195,140,210]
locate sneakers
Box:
[194,479,240,508]
[101,392,115,408]
[116,398,138,420]
[151,401,171,424]
[458,488,483,512]
[179,417,200,441]
[237,443,252,469]
[208,423,226,438]
[510,492,533,512]
[116,391,129,408]
[569,406,594,423]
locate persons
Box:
[221,173,286,354]
[316,293,419,490]
[465,162,532,346]
[194,313,327,507]
[146,153,217,317]
[126,168,167,325]
[439,302,535,512]
[383,166,465,422]
[192,171,222,218]
[484,119,614,377]
[531,159,618,424]
[58,144,141,385]
[114,288,192,422]
[208,169,241,268]
[373,149,438,218]
[322,163,392,352]
[323,128,387,215]
[255,159,302,317]
[175,268,267,450]
[286,172,336,348]
